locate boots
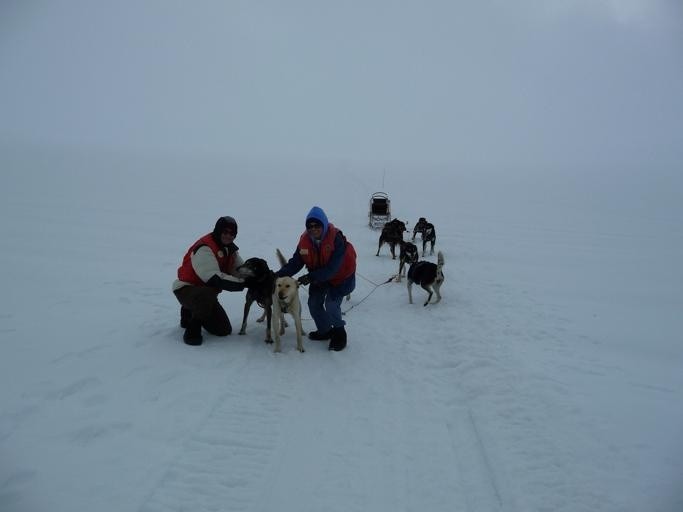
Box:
[327,326,346,351]
[308,328,331,340]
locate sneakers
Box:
[182,329,202,345]
[180,306,193,328]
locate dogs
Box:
[413,218,436,258]
[376,218,407,260]
[270,249,308,354]
[234,257,279,344]
[407,250,446,307]
[397,239,419,283]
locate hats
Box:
[305,205,329,241]
[214,216,238,239]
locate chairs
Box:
[367,191,391,230]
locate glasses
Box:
[305,223,321,229]
[222,229,235,236]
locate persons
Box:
[276,205,357,351]
[173,216,248,346]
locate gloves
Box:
[246,278,264,288]
[297,273,311,285]
